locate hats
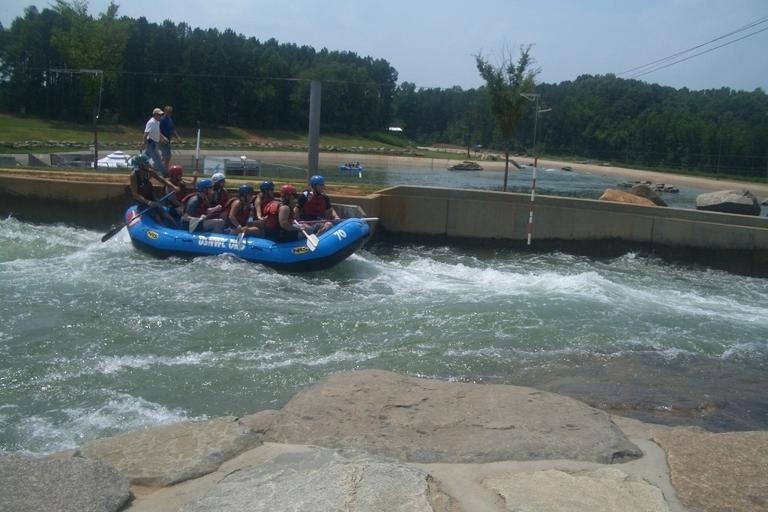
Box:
[152,108,163,114]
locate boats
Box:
[447,161,483,171]
[339,163,364,173]
[561,165,575,171]
[124,203,372,272]
[86,148,156,170]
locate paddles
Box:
[297,217,379,224]
[237,201,254,245]
[101,191,176,242]
[293,218,319,252]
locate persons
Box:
[343,160,360,168]
[140,107,172,179]
[132,154,341,246]
[159,105,183,170]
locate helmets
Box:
[132,154,324,194]
